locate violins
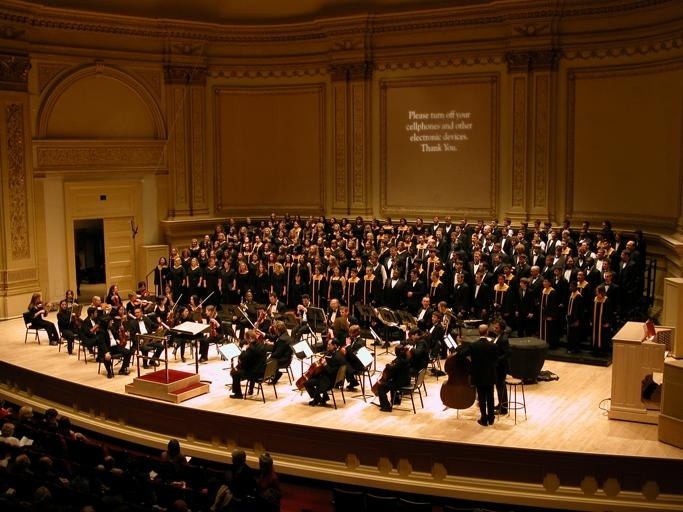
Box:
[35,290,217,347]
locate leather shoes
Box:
[347,380,360,390]
[309,396,323,405]
[230,393,243,399]
[48,340,65,346]
[107,360,160,378]
[320,395,331,403]
[477,404,508,426]
[268,372,283,385]
[439,350,448,360]
[377,398,402,412]
[371,340,391,349]
[172,348,209,363]
[247,389,254,396]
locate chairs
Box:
[333,488,492,511]
[0,406,282,511]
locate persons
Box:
[0,395,278,508]
[151,210,649,426]
[27,280,163,377]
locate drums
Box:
[505,337,548,384]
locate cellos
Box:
[441,320,476,409]
[296,346,342,391]
[371,345,415,396]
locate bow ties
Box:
[271,303,276,305]
[422,307,427,311]
[303,304,308,307]
[140,318,144,322]
[372,223,627,292]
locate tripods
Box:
[224,359,246,392]
[352,372,374,402]
[377,330,394,357]
[290,366,308,398]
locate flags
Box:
[644,320,656,339]
